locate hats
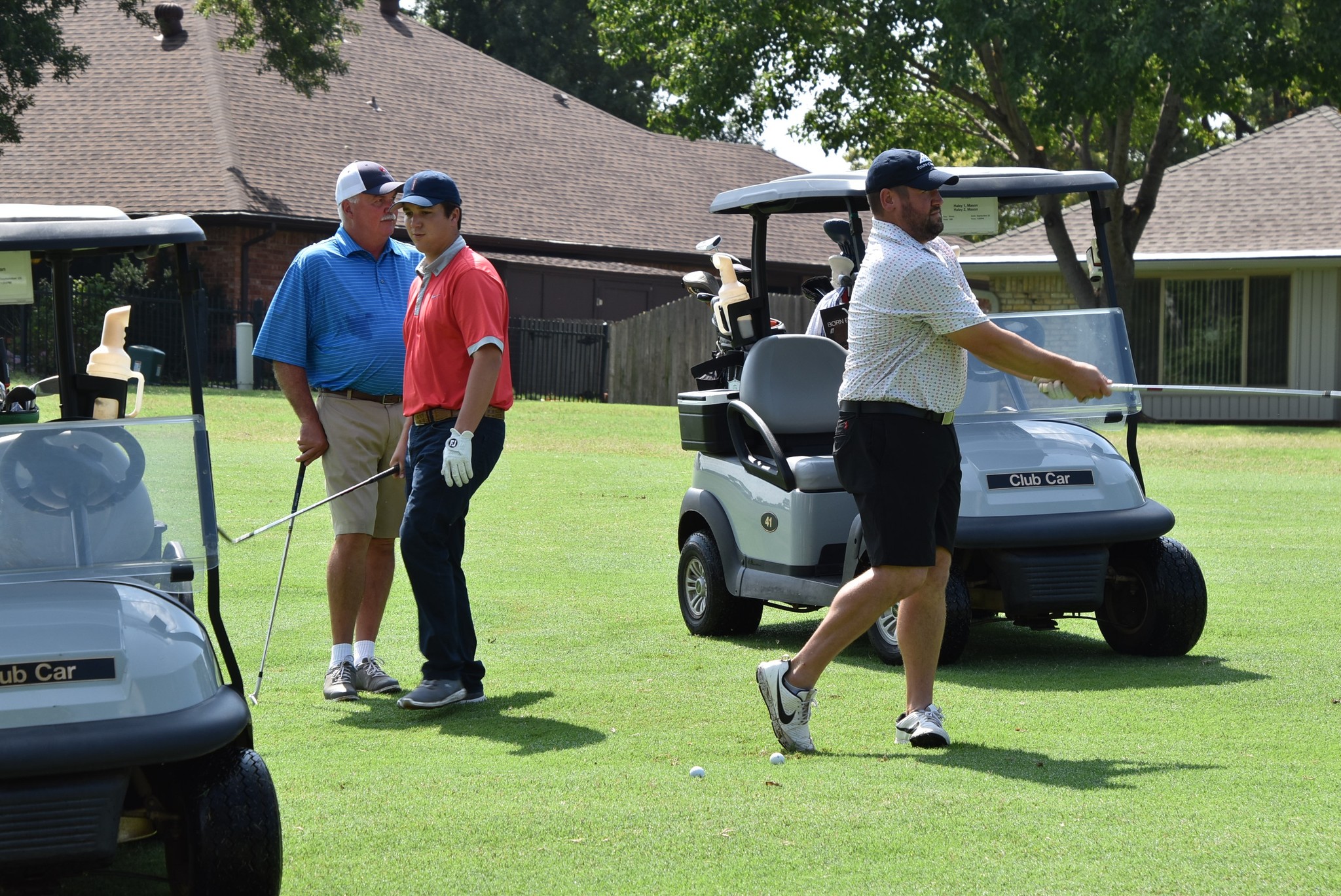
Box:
[335,160,406,205]
[389,170,461,212]
[865,148,959,195]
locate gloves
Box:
[440,428,474,487]
[1032,377,1075,400]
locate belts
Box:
[320,386,403,405]
[839,400,955,426]
[413,405,504,427]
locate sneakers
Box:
[397,677,466,710]
[448,690,488,705]
[756,654,818,753]
[323,655,360,701]
[355,658,402,693]
[894,704,950,747]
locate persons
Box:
[751,148,1114,752]
[270,160,426,703]
[388,169,513,709]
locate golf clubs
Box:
[217,465,399,546]
[248,451,308,706]
[1038,380,1341,399]
[694,235,722,253]
[710,251,741,265]
[0,375,59,411]
